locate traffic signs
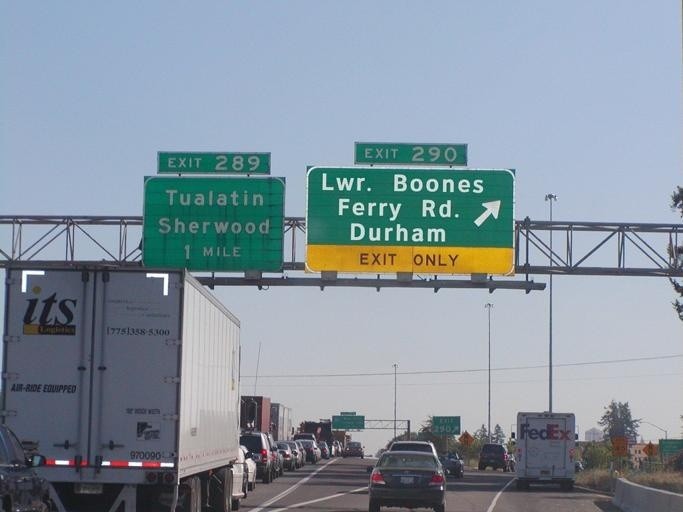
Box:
[352,141,470,168]
[153,150,274,176]
[140,175,287,275]
[303,165,516,277]
[431,416,460,436]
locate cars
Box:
[231,430,345,512]
[365,439,464,512]
[344,441,364,460]
[476,443,516,475]
[574,458,585,474]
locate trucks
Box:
[238,392,294,443]
[0,262,243,512]
[511,412,580,490]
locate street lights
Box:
[483,301,494,444]
[391,361,397,439]
[541,191,557,410]
